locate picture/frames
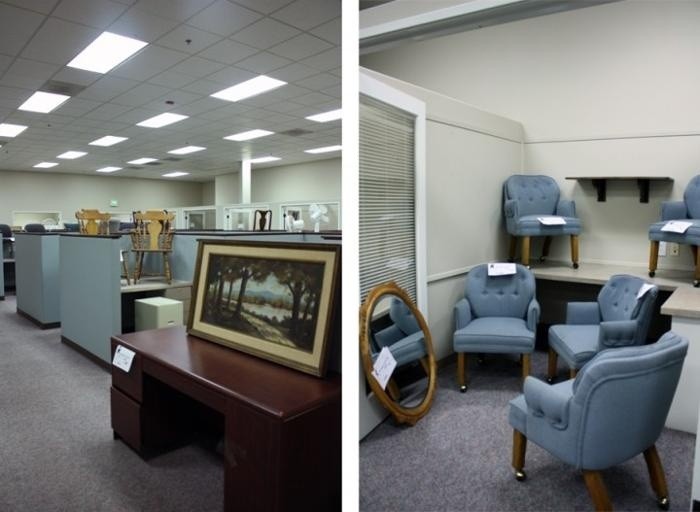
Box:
[187,238,340,378]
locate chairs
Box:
[74,208,177,285]
[510,330,689,511]
[546,275,676,386]
[648,171,699,288]
[370,294,430,401]
[503,175,582,267]
[452,263,541,393]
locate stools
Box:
[134,297,183,332]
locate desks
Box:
[111,325,341,509]
[513,259,700,436]
[3,224,340,373]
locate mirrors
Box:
[359,279,437,426]
